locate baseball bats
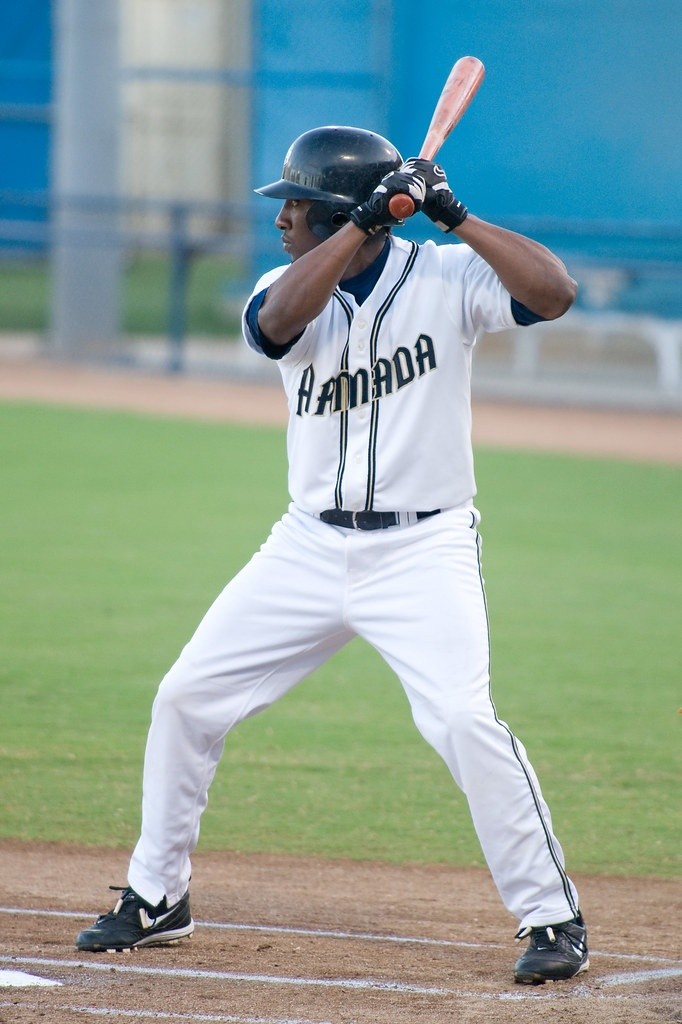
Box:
[384,53,486,218]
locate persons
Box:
[78,123,589,986]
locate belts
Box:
[319,506,441,533]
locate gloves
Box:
[349,170,426,238]
[399,156,468,234]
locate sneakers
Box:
[512,905,590,984]
[75,885,196,954]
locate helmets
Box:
[253,125,404,242]
